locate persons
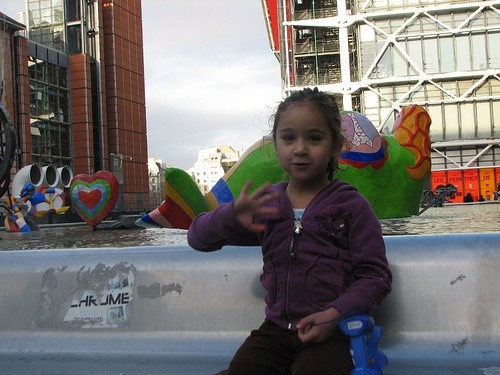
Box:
[464,192,474,202]
[479,195,485,201]
[187,86,393,375]
